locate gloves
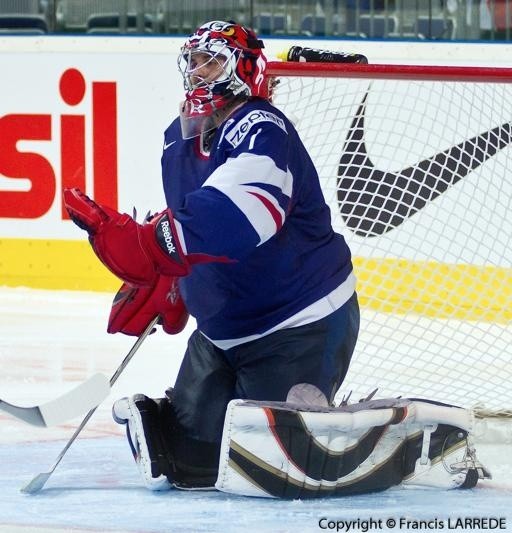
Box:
[63,187,239,336]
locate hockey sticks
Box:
[1,374,110,427]
[20,316,159,492]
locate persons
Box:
[62,19,493,490]
[435,0,495,41]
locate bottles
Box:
[277,45,370,64]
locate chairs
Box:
[356,15,398,39]
[300,16,338,35]
[86,13,155,35]
[480,28,512,41]
[332,1,396,32]
[414,16,453,39]
[251,13,292,36]
[0,14,49,35]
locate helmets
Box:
[178,20,268,140]
